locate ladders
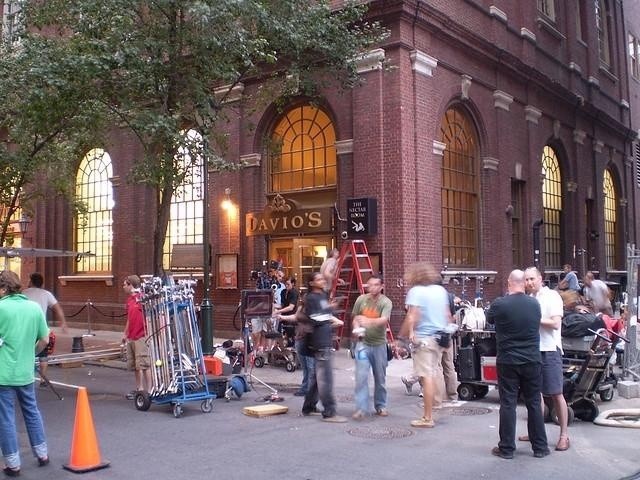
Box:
[330,239,400,360]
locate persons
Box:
[583,272,613,317]
[248,260,278,364]
[558,264,581,290]
[278,289,317,397]
[487,269,552,459]
[0,269,49,476]
[553,273,567,290]
[275,274,298,336]
[274,270,286,332]
[122,275,152,400]
[439,293,459,403]
[320,248,345,292]
[401,304,427,397]
[393,261,446,428]
[301,273,349,422]
[22,273,69,387]
[350,275,393,419]
[519,267,570,451]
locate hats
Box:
[128,274,141,289]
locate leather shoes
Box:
[520,435,530,440]
[491,447,513,459]
[554,439,570,451]
[534,448,550,458]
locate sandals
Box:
[3,464,20,476]
[39,455,49,465]
[127,390,142,400]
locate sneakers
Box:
[40,382,47,385]
[377,408,388,416]
[352,410,368,420]
[322,414,347,422]
[294,391,304,396]
[410,417,434,427]
[303,407,324,415]
[452,399,456,403]
[420,400,442,410]
[401,375,413,395]
[419,387,423,397]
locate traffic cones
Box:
[63,387,111,473]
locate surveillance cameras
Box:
[341,231,348,240]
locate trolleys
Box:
[561,329,616,402]
[544,329,630,425]
[453,307,527,403]
[134,273,217,417]
